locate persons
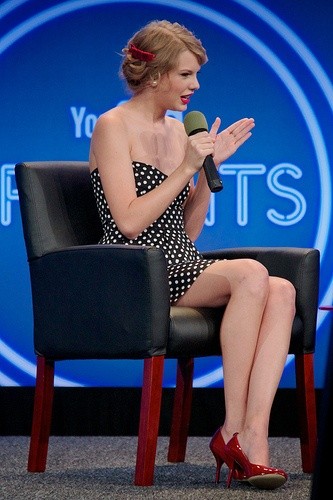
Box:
[89,19,297,490]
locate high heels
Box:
[210,426,249,486]
[224,432,288,489]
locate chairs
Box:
[16,161,320,487]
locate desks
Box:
[310,306,333,500]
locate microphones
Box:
[183,110,223,193]
